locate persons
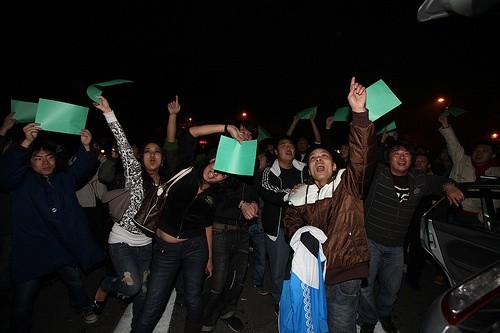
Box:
[0,110,500,333]
[93,95,170,333]
[282,76,375,332]
[137,122,243,333]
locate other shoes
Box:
[251,283,269,296]
[92,277,107,315]
[360,323,375,333]
[433,275,444,284]
[405,277,422,290]
[79,310,98,324]
[380,315,397,333]
[220,315,244,333]
[274,305,279,316]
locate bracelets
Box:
[224,124,228,133]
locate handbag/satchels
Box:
[102,174,143,222]
[133,166,195,233]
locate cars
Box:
[418,176,500,333]
